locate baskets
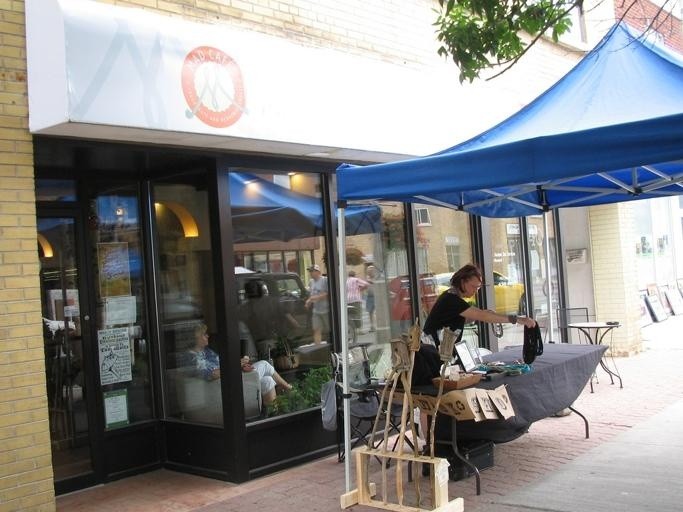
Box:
[275,337,301,369]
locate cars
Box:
[433,269,526,327]
[388,272,436,322]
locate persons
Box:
[409,264,537,442]
[182,322,293,417]
[346,270,369,344]
[303,264,331,345]
[365,265,377,332]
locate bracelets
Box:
[511,315,517,324]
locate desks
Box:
[362,342,610,496]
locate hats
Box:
[307,264,321,271]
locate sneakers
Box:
[358,326,376,335]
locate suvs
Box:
[233,266,363,383]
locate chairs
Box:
[154,363,265,426]
[326,343,419,470]
[557,308,599,385]
